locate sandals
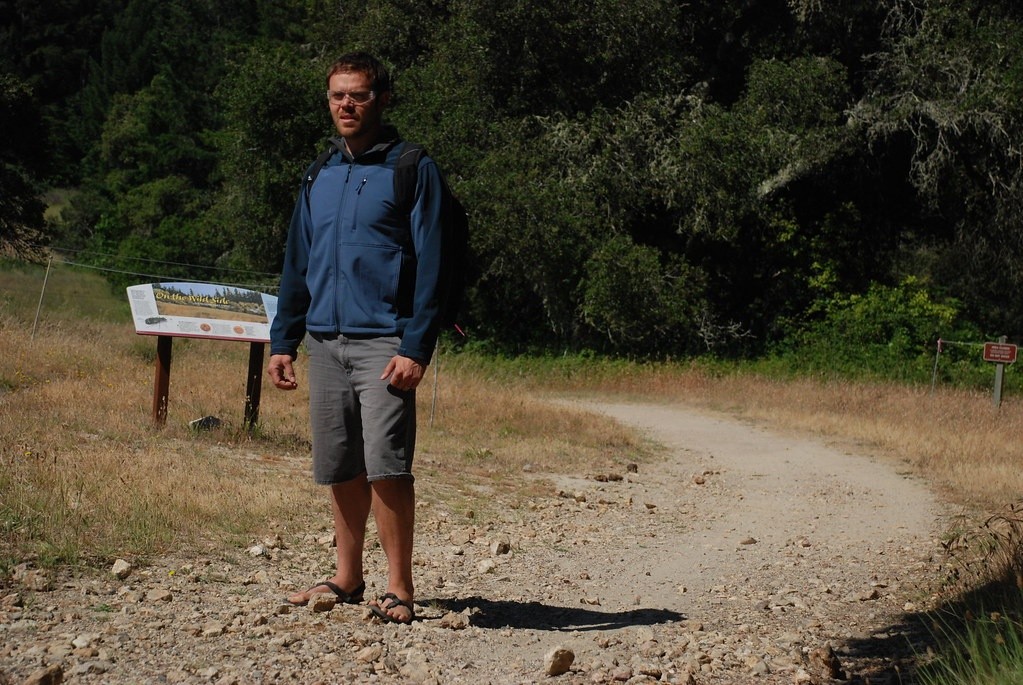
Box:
[283,580,366,607]
[370,593,415,624]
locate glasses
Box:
[327,88,377,106]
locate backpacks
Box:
[302,141,472,330]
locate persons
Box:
[269,50,449,623]
